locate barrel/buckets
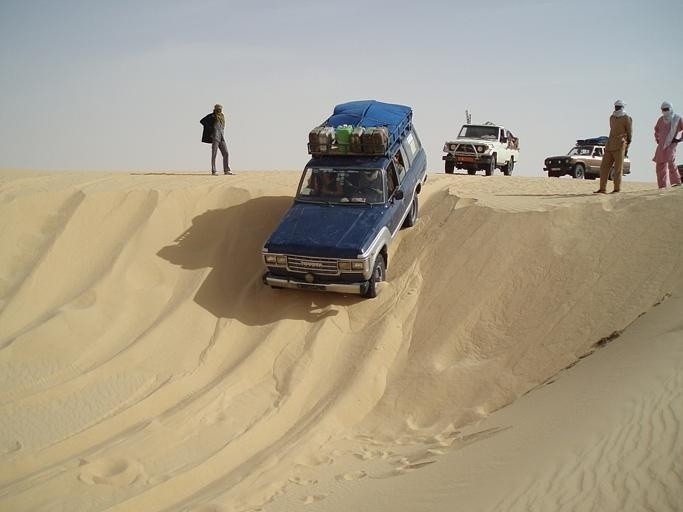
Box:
[335,124,354,153]
[351,125,366,154]
[309,126,324,158]
[318,124,335,152]
[371,124,389,154]
[361,125,376,153]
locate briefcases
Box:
[309,124,387,153]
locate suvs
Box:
[544,143,630,180]
[259,100,427,299]
[442,124,518,174]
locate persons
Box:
[200,104,234,177]
[652,101,683,188]
[593,99,633,194]
[358,171,395,203]
